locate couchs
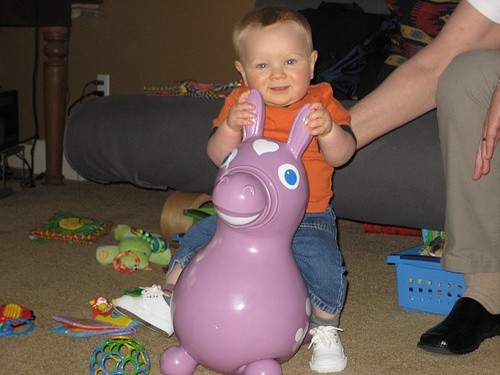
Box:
[63,0,445,229]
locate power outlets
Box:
[96,72,110,94]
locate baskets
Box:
[385,243,468,315]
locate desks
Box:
[0,0,102,187]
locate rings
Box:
[482,138,486,141]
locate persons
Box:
[348,0,500,355]
[111,5,357,373]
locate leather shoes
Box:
[417,297,500,356]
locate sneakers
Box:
[110,284,174,338]
[308,325,348,373]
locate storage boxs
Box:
[386,243,464,316]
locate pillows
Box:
[384,0,457,79]
